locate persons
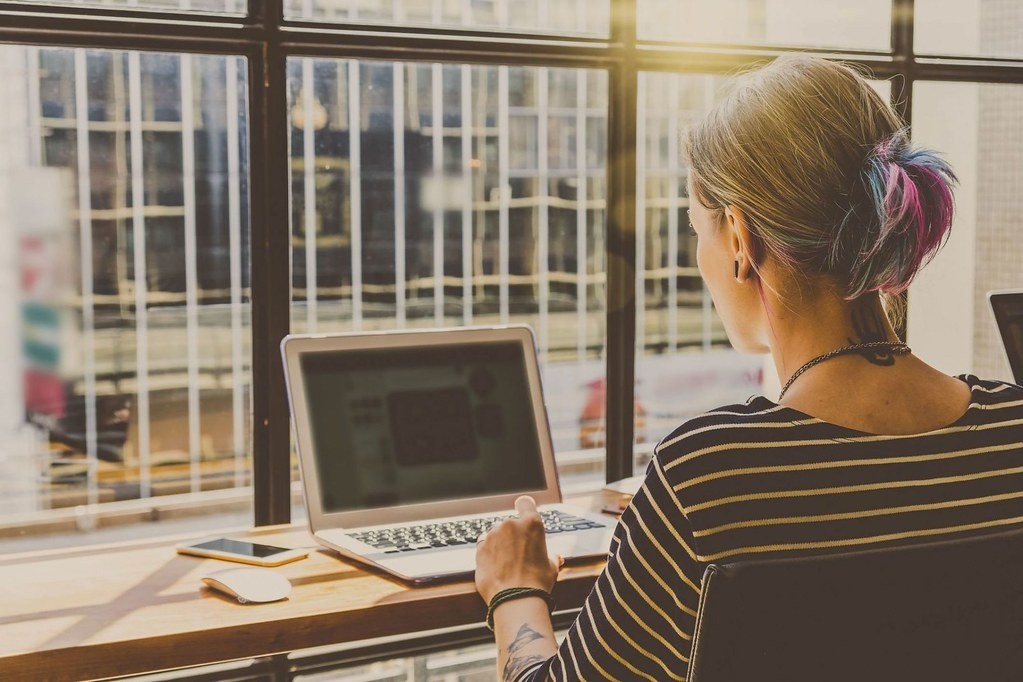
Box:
[474,52,1023,682]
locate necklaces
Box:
[777,341,912,403]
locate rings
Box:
[476,535,485,545]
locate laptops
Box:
[281,323,619,586]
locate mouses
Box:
[200,566,292,603]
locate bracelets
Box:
[485,587,556,632]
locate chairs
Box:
[683,527,1023,682]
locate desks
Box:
[0,493,634,682]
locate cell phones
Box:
[176,537,309,567]
